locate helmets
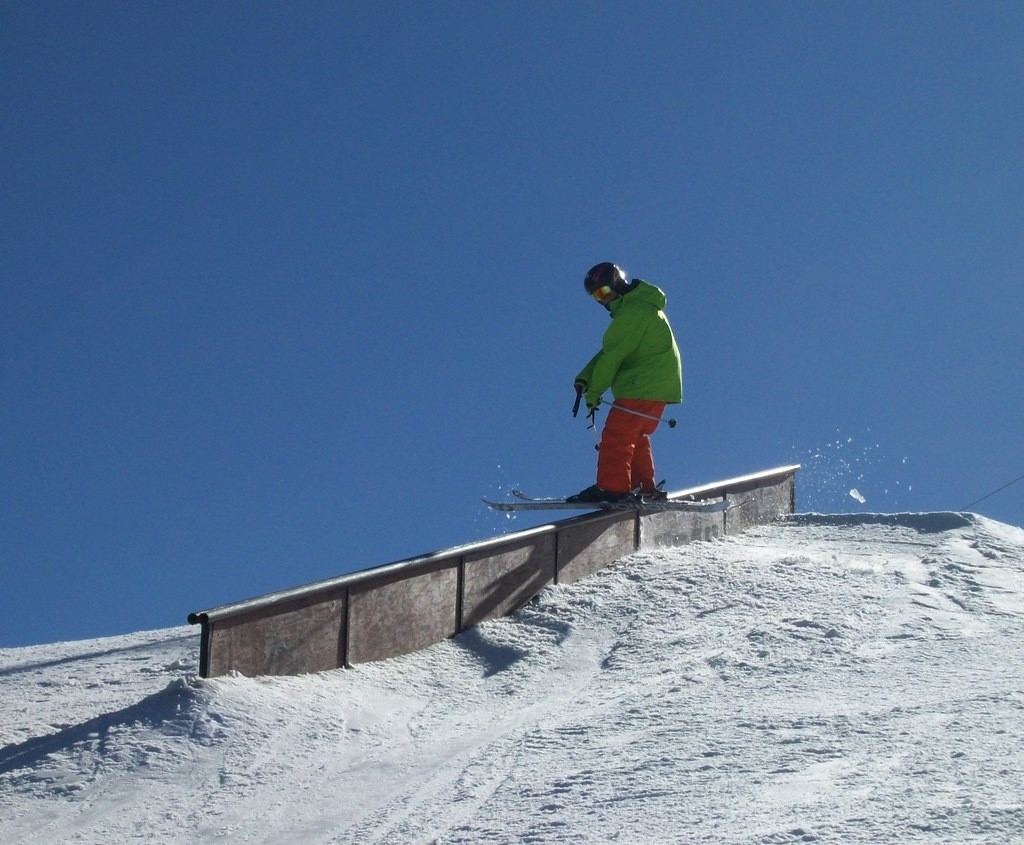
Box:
[584,262,626,311]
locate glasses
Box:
[592,284,613,302]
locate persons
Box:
[566,262,683,503]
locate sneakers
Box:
[565,484,632,504]
[634,486,668,501]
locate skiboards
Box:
[480,491,755,512]
